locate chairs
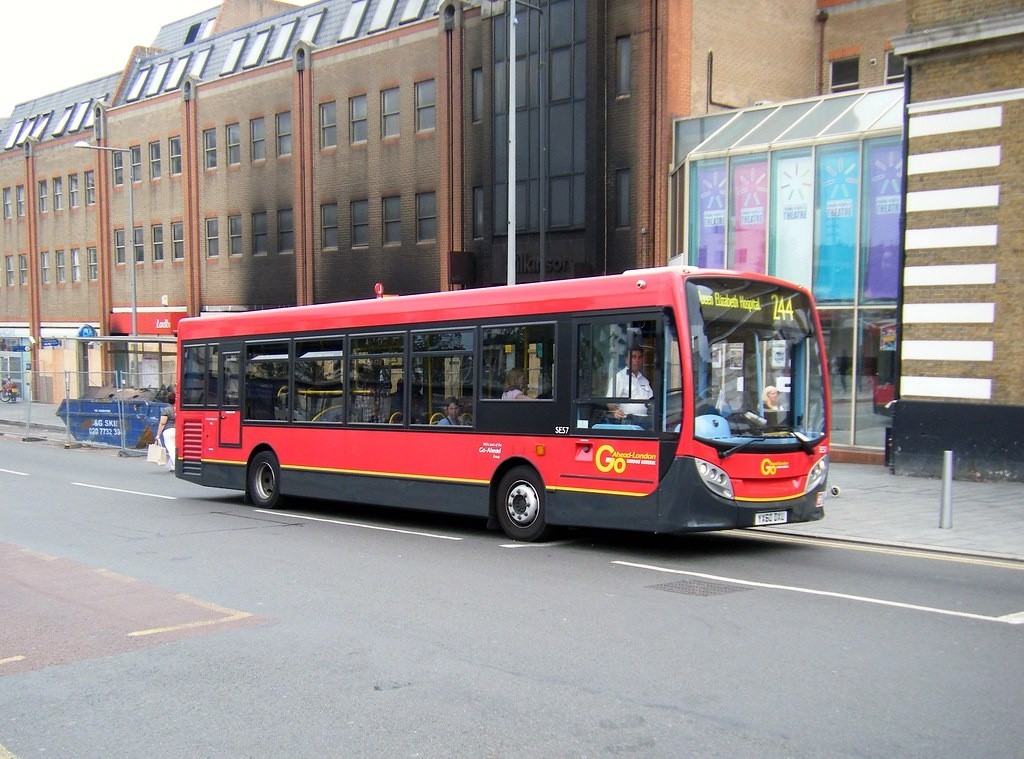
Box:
[187,369,609,427]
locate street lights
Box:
[73,140,139,389]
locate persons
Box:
[501,366,539,399]
[604,343,655,419]
[1,377,18,402]
[154,392,176,472]
[835,348,849,394]
[223,346,473,428]
[760,385,786,412]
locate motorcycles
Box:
[0,379,18,402]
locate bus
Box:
[174,265,836,544]
[869,318,897,418]
